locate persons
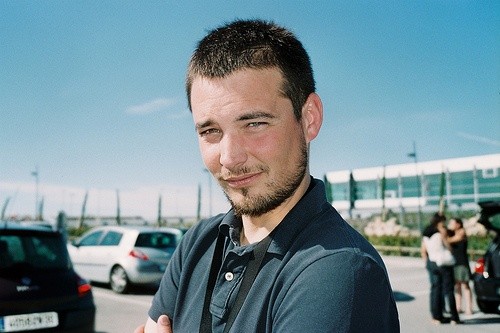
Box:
[132,19,400,333]
[421,212,473,326]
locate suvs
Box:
[471,197,500,313]
[67,223,190,294]
[0,221,95,333]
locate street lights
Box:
[31,172,38,220]
[408,154,421,236]
[203,168,212,217]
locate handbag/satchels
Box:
[434,250,456,267]
